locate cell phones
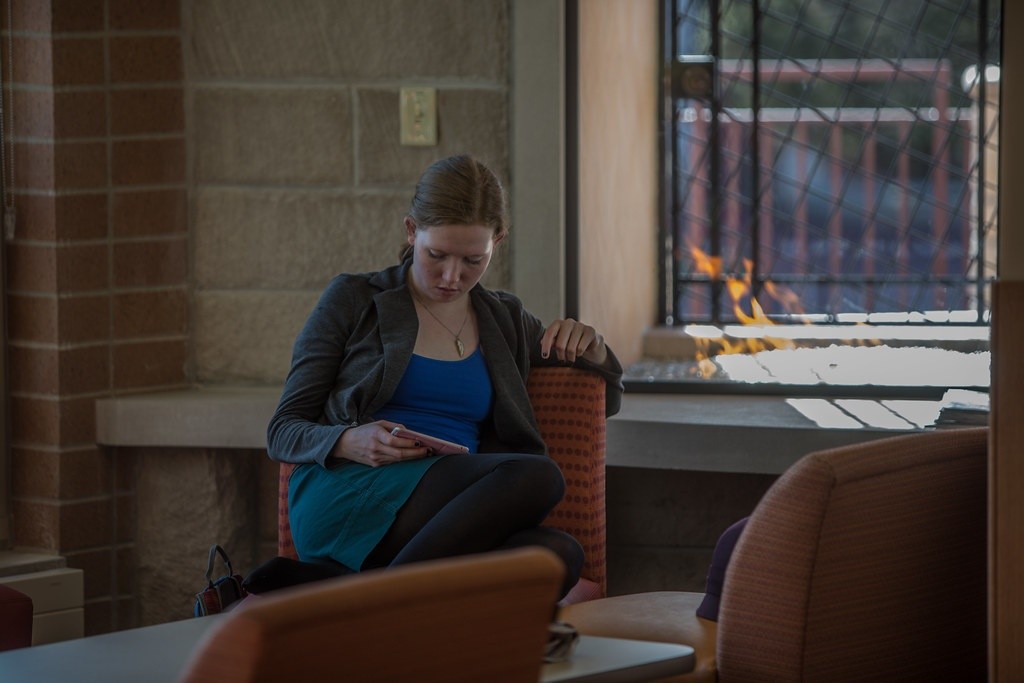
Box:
[390,426,470,457]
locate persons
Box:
[266,152,624,606]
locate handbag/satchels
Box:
[192,544,248,619]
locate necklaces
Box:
[413,294,471,357]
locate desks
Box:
[0,610,699,682]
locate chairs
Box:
[278,358,608,608]
[556,424,990,683]
[175,545,565,683]
